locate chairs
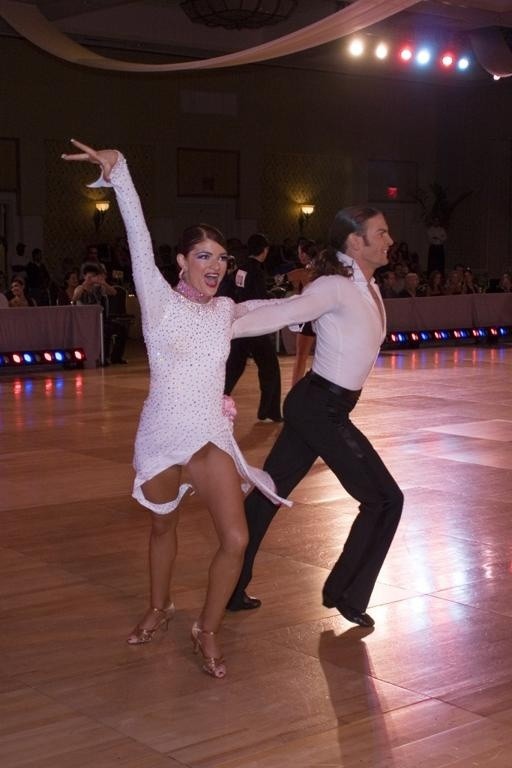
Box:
[106,285,137,345]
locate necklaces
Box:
[174,276,209,302]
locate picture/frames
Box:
[366,159,418,204]
[176,147,241,199]
[0,137,21,193]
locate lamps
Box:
[92,200,112,234]
[299,204,315,229]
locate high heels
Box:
[192,621,227,678]
[128,602,175,644]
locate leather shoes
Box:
[257,410,284,422]
[112,357,127,364]
[227,591,260,610]
[103,359,109,366]
[322,590,375,627]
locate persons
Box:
[227,199,409,630]
[265,237,321,387]
[56,137,316,681]
[372,212,511,298]
[214,232,285,423]
[1,212,138,368]
[149,236,328,299]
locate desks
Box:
[1,304,104,370]
[275,294,512,356]
